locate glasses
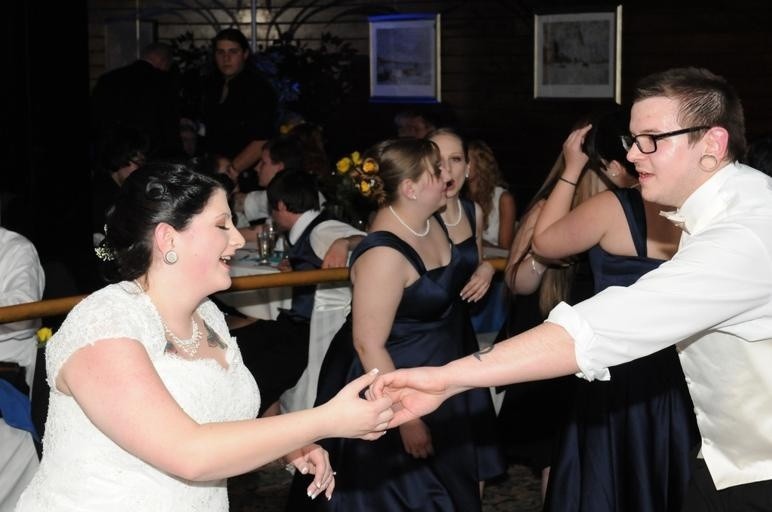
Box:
[619,124,714,155]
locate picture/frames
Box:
[365,12,444,104]
[528,4,625,108]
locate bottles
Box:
[252,219,287,260]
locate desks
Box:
[217,236,299,320]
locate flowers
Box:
[334,150,383,202]
